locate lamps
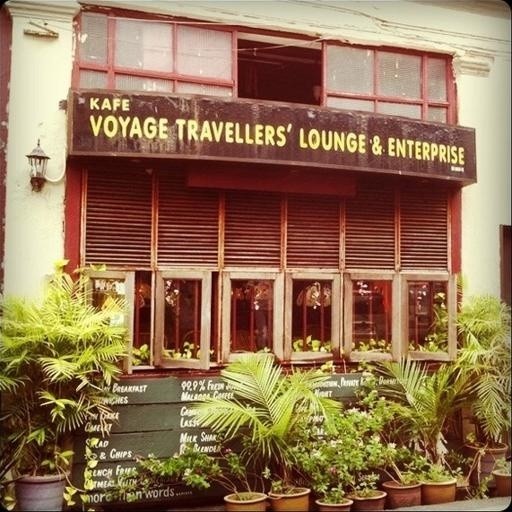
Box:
[26,140,49,192]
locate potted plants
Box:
[224,492,268,511]
[492,469,512,497]
[349,490,388,511]
[0,261,148,512]
[315,498,354,512]
[419,477,457,504]
[383,480,423,506]
[464,441,508,485]
[269,488,311,511]
